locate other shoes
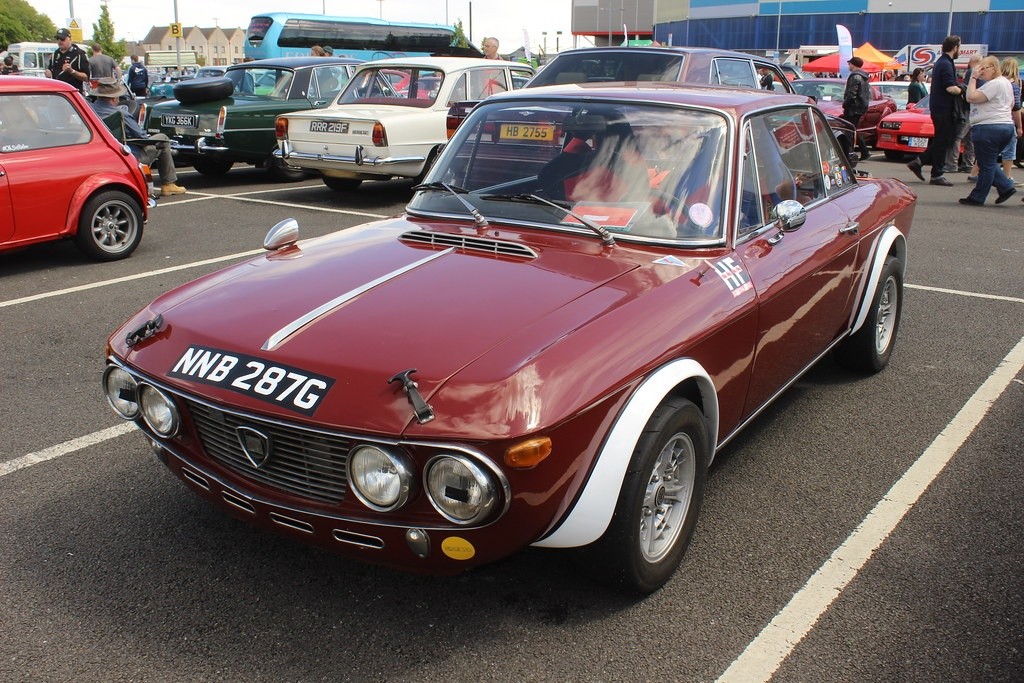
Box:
[957,167,972,173]
[958,196,984,206]
[858,151,872,161]
[929,177,953,186]
[1007,180,1014,186]
[968,175,978,181]
[907,160,925,181]
[995,187,1016,204]
[943,165,958,173]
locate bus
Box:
[244,13,458,66]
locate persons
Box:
[886,54,1024,206]
[907,36,968,186]
[842,57,872,160]
[236,55,256,96]
[476,37,508,100]
[89,77,187,197]
[311,46,353,96]
[0,56,21,76]
[755,66,776,92]
[126,54,149,97]
[44,28,91,96]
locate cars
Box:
[925,64,968,85]
[450,46,850,196]
[779,80,896,149]
[103,81,918,595]
[273,59,538,189]
[763,64,819,89]
[90,59,441,176]
[874,94,964,159]
[0,76,156,260]
[866,81,932,108]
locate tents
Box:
[802,42,901,82]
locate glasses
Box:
[848,63,850,65]
[981,65,994,70]
[56,37,65,41]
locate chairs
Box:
[555,72,588,83]
[638,74,668,81]
[536,111,648,219]
[665,118,800,236]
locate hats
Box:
[323,45,333,54]
[54,28,71,37]
[847,56,864,68]
[89,77,125,97]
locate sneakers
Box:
[161,184,186,195]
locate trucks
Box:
[7,42,92,75]
